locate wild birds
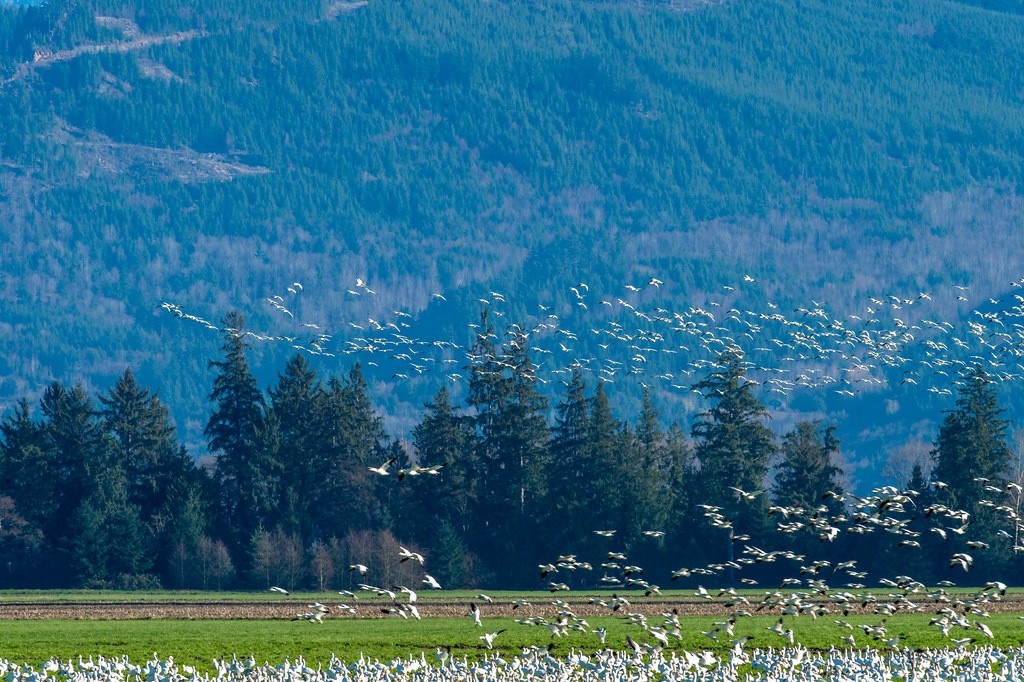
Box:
[367,457,449,482]
[1,473,1023,682]
[160,269,1024,401]
